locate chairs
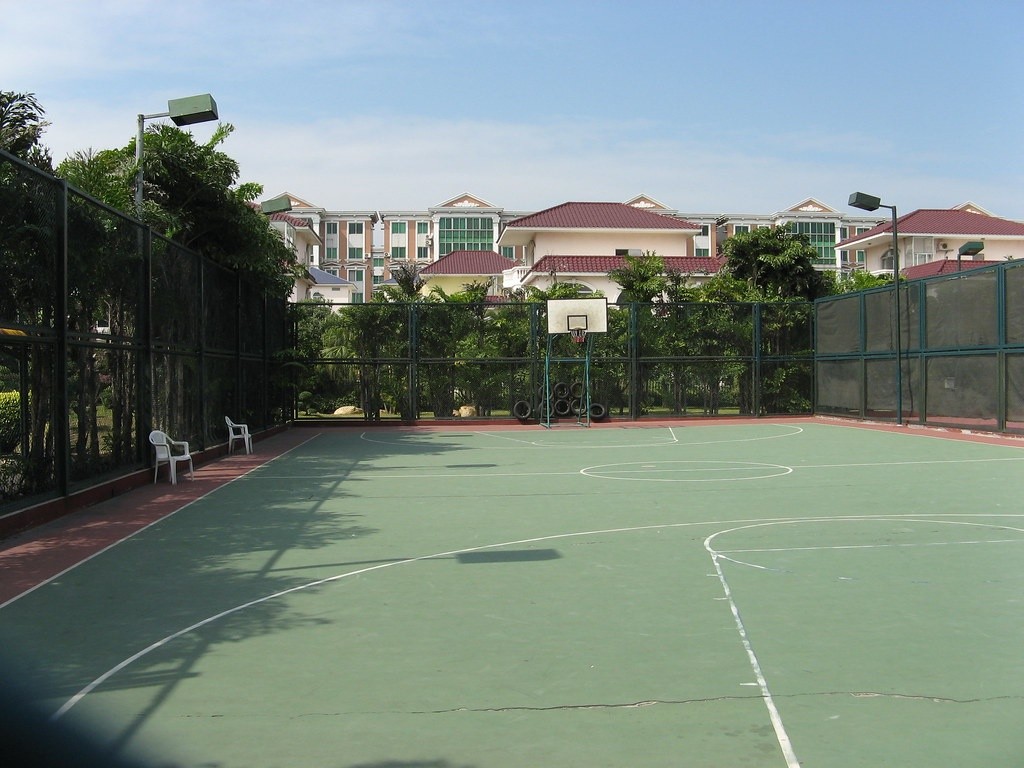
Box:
[225,416,253,455]
[149,430,195,486]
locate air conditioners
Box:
[425,234,433,239]
[364,253,372,259]
[425,240,432,246]
[937,242,949,251]
[384,252,391,258]
